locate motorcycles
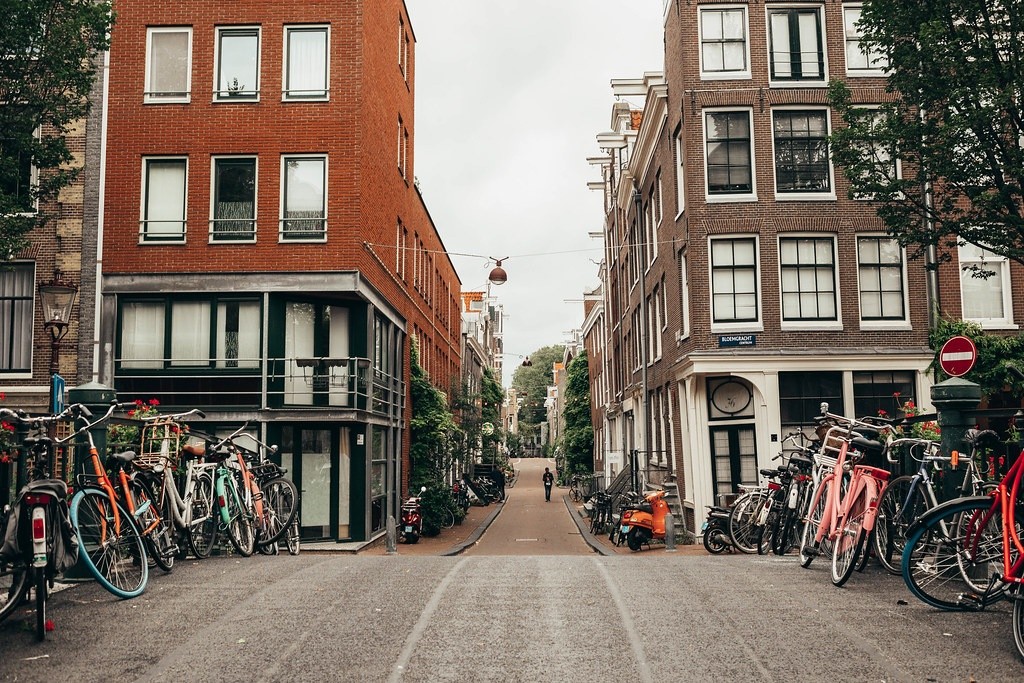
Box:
[453,483,471,514]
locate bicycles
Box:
[699,402,1024,660]
[569,475,647,548]
[473,475,503,504]
[439,493,455,530]
[0,399,301,644]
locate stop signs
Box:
[938,335,977,376]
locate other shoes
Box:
[546,500,550,502]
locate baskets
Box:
[815,417,874,449]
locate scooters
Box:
[620,489,671,552]
[397,485,428,543]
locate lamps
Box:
[489,257,508,285]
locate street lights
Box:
[37,268,79,416]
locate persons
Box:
[543,467,554,502]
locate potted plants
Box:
[925,311,1024,450]
[581,474,593,503]
